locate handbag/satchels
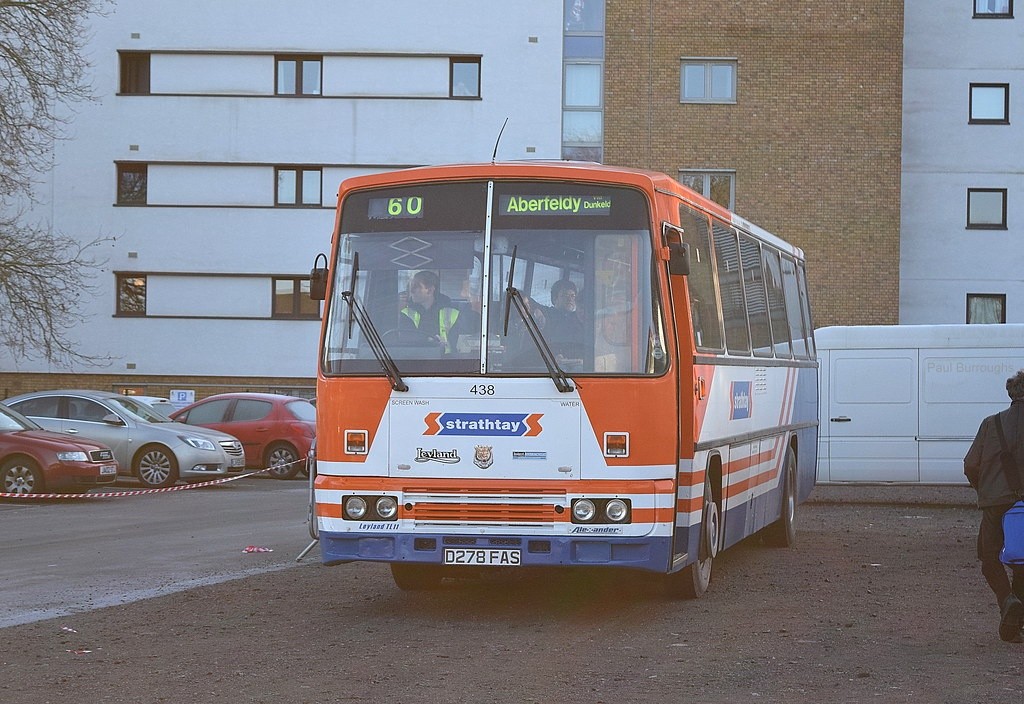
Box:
[998,500,1024,566]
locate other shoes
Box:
[999,592,1024,641]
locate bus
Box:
[307,159,819,597]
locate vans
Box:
[753,325,1023,489]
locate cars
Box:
[0,390,247,491]
[163,392,318,479]
[104,395,179,423]
[0,403,121,505]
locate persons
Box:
[400,270,467,355]
[498,251,640,372]
[964,371,1024,642]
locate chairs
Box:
[68,404,84,420]
[83,403,101,421]
